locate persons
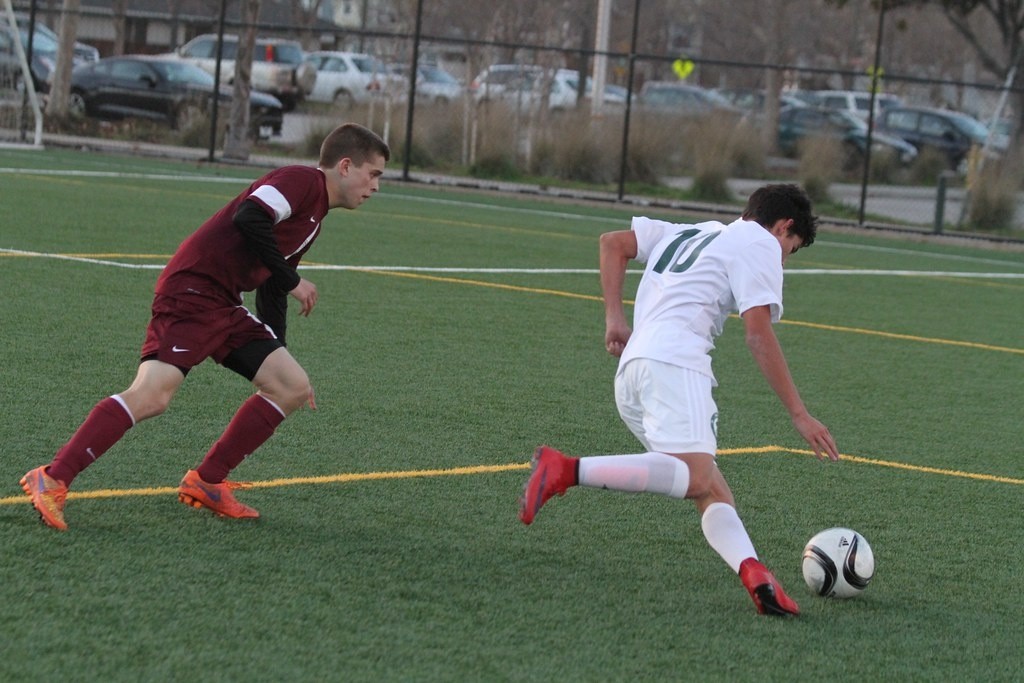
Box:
[520,182,839,620]
[21,123,391,532]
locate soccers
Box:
[802,527,876,599]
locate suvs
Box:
[806,91,903,126]
[475,65,626,117]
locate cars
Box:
[303,52,413,111]
[772,108,916,171]
[603,82,807,125]
[0,14,101,95]
[385,61,465,113]
[45,54,284,140]
[870,107,1008,168]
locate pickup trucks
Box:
[167,31,318,114]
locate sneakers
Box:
[19,464,68,530]
[519,446,568,525]
[739,567,799,618]
[178,469,259,519]
